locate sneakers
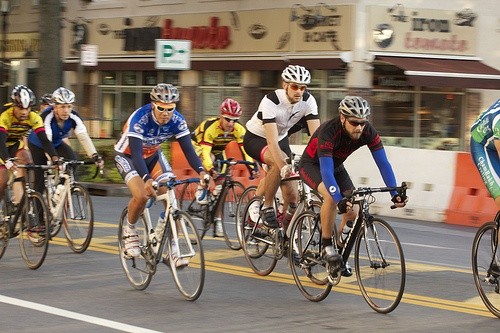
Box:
[162,251,187,270]
[258,202,279,228]
[123,228,142,257]
[279,241,300,262]
[319,239,342,263]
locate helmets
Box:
[51,87,76,104]
[281,65,312,85]
[338,96,371,119]
[40,94,53,105]
[11,84,37,108]
[219,98,242,117]
[150,84,180,103]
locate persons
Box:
[296,96,405,277]
[29,88,105,240]
[38,93,54,115]
[469,96,500,210]
[190,97,259,238]
[113,84,214,266]
[243,64,320,262]
[0,85,61,245]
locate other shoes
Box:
[195,185,208,204]
[248,200,263,223]
[213,217,226,237]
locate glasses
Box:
[151,102,176,113]
[287,82,305,91]
[345,117,368,127]
[224,118,238,123]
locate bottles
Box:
[155,211,166,237]
[210,185,223,200]
[54,183,63,201]
[339,221,353,244]
[282,202,298,226]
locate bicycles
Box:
[0,154,102,269]
[177,157,257,250]
[117,174,211,302]
[235,152,408,314]
[470,210,500,318]
